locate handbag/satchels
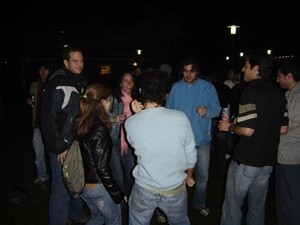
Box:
[63,143,84,195]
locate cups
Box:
[222,108,230,123]
[31,96,36,109]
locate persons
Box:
[40,45,85,225]
[25,52,300,225]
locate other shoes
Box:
[156,209,165,223]
[200,208,208,216]
[35,175,48,183]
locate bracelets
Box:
[229,124,236,134]
